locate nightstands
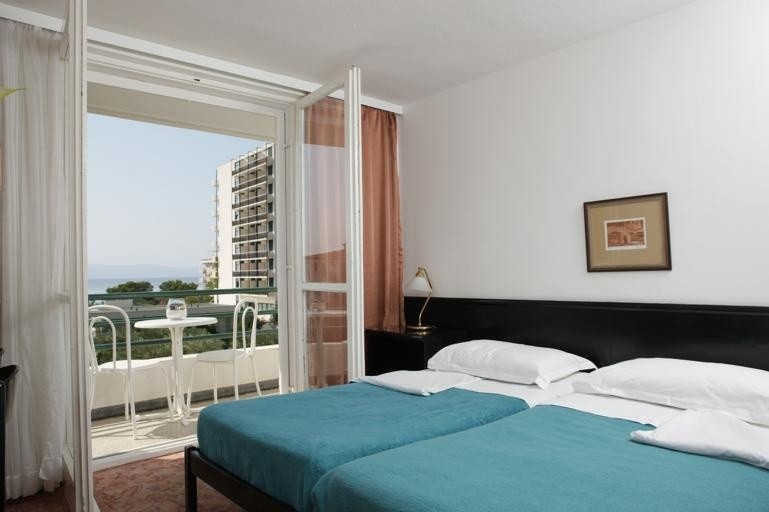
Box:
[364,329,442,377]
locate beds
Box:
[183,369,590,512]
[309,392,769,510]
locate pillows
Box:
[426,338,598,390]
[574,357,769,428]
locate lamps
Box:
[402,263,435,336]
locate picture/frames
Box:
[579,191,674,275]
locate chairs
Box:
[85,297,267,440]
